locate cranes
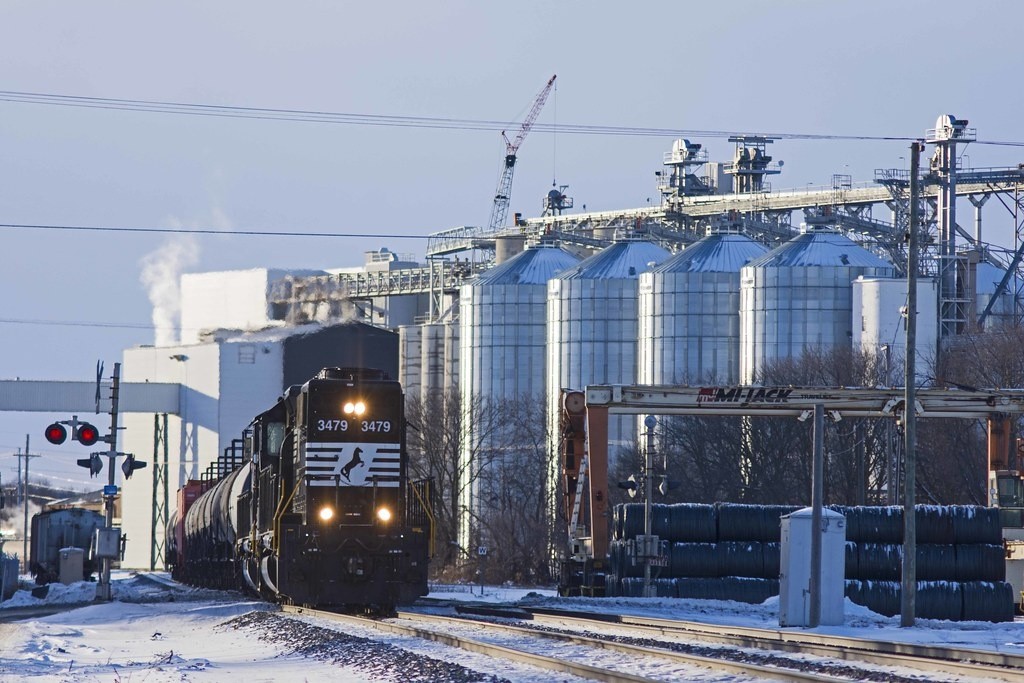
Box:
[421,72,561,325]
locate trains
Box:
[30,507,101,583]
[167,366,433,618]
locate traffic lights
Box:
[123,456,147,480]
[77,452,94,479]
[78,424,99,447]
[44,424,67,445]
